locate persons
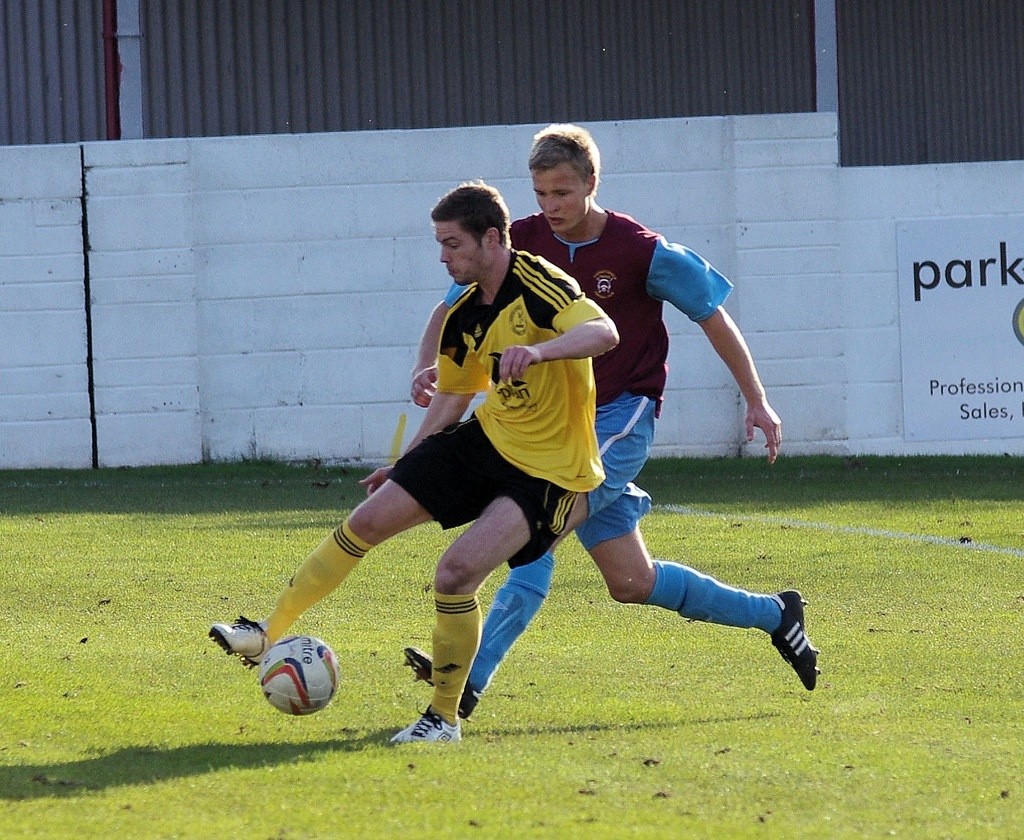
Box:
[207,176,619,744]
[406,123,822,719]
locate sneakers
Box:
[387,702,463,749]
[208,615,268,672]
[770,589,822,691]
[402,647,480,720]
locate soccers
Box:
[260,635,341,716]
